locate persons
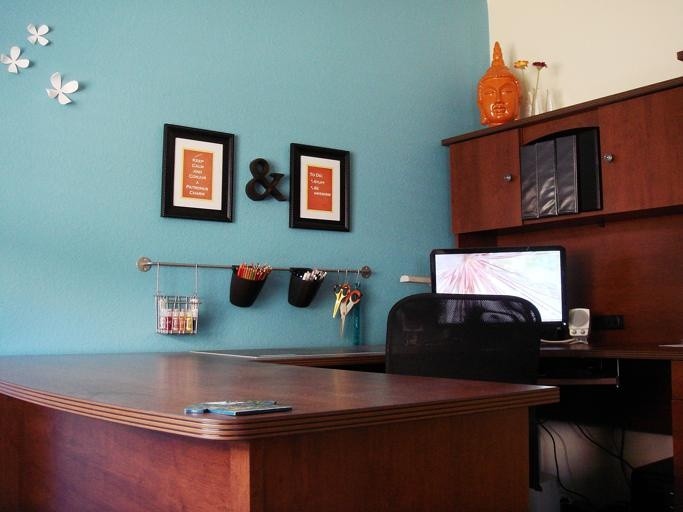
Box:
[477,41,524,129]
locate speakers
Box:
[568,308,591,345]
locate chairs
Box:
[384,294,542,386]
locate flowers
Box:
[512,60,547,116]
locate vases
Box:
[523,89,544,118]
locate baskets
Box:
[156,296,200,335]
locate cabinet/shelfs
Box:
[439,74,683,344]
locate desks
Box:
[0,343,683,512]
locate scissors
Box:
[332,283,362,336]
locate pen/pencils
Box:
[236,263,272,281]
[299,269,328,281]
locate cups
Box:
[230,264,268,308]
[287,267,323,308]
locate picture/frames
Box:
[159,123,234,224]
[288,142,351,231]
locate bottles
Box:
[160,298,199,334]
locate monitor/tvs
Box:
[430,245,568,331]
